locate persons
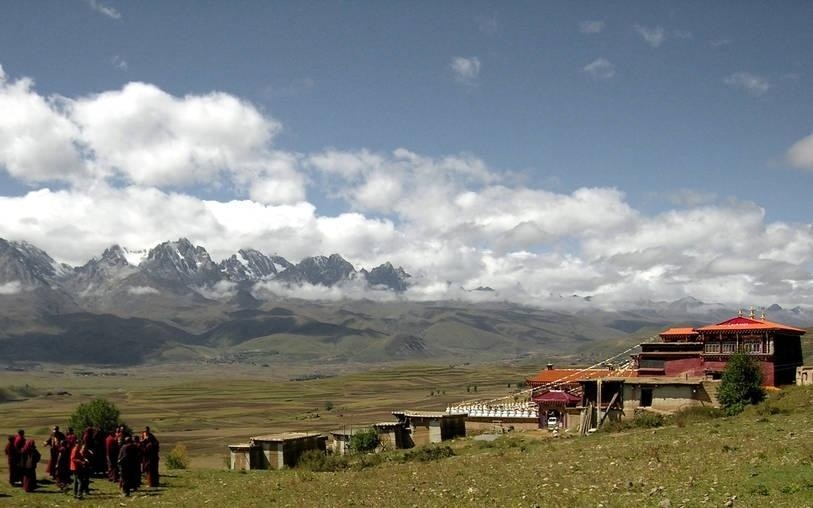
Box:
[2,422,159,500]
